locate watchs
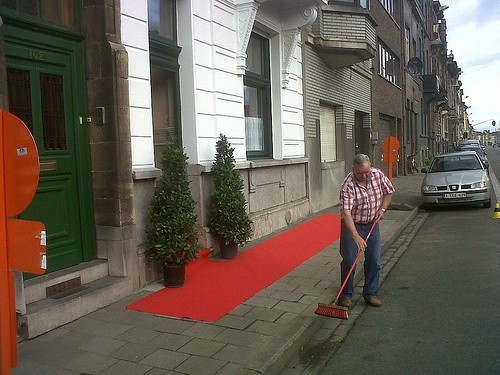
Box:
[379,207,386,213]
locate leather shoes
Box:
[342,296,351,306]
[365,295,382,307]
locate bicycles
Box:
[407,151,419,174]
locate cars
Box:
[421,150,492,208]
[458,139,500,177]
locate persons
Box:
[340,153,396,306]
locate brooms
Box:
[313,219,377,320]
